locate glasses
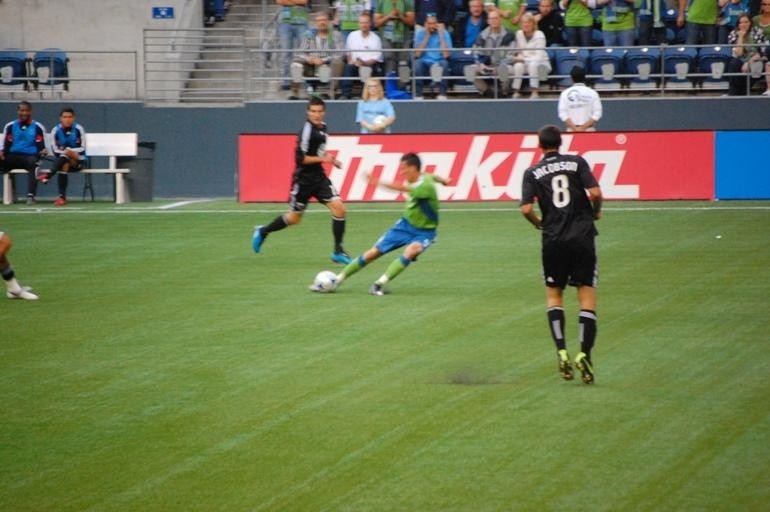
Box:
[426,13,437,17]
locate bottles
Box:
[305,81,313,101]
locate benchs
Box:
[0,133,139,205]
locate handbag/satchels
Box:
[511,92,523,97]
[478,55,491,65]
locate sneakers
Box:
[328,252,352,265]
[26,197,35,205]
[288,87,298,99]
[762,89,770,96]
[55,196,66,206]
[436,95,447,99]
[484,89,494,99]
[308,284,317,289]
[368,283,384,296]
[252,225,265,253]
[557,349,574,380]
[7,290,38,300]
[530,92,539,98]
[36,174,50,185]
[574,351,594,384]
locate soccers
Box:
[314,271,337,293]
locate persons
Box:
[354,82,396,134]
[562,0,719,45]
[34,107,86,205]
[271,1,563,100]
[309,152,438,296]
[253,97,354,265]
[0,230,39,301]
[0,102,46,205]
[558,66,604,133]
[720,0,770,97]
[521,126,602,384]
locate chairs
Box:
[662,9,679,26]
[1,48,30,91]
[449,46,732,93]
[31,47,69,90]
